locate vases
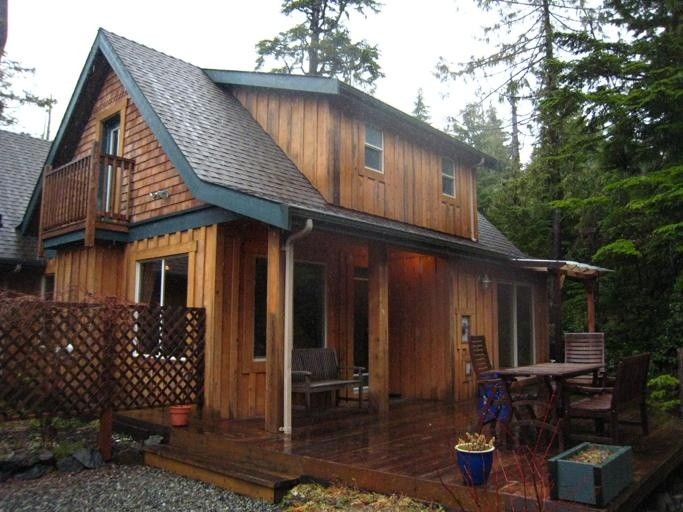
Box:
[169,406,191,426]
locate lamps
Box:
[480,272,491,289]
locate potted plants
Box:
[547,441,632,508]
[455,431,497,486]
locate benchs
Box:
[290,347,366,412]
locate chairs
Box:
[468,331,652,453]
